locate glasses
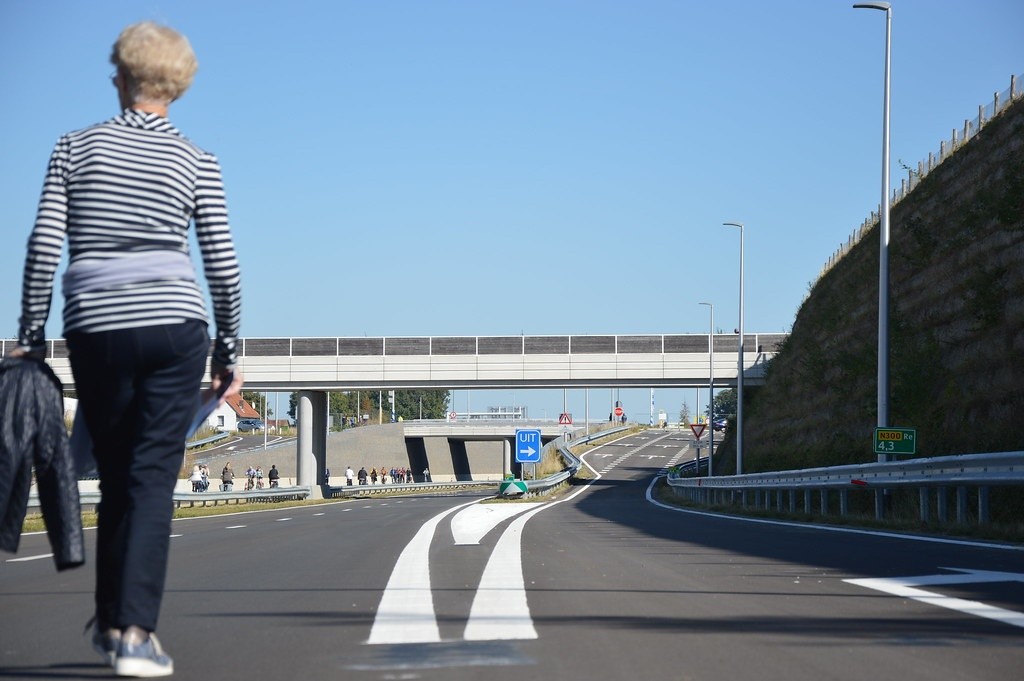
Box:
[109,68,120,87]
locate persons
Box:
[255,466,264,482]
[9,22,240,677]
[390,467,412,483]
[246,465,257,483]
[221,462,235,486]
[358,467,367,485]
[345,467,354,485]
[381,467,387,477]
[190,464,209,491]
[370,467,378,482]
[269,465,279,486]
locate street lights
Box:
[698,301,714,478]
[851,1,892,498]
[721,221,744,494]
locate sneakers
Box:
[84,616,124,666]
[114,625,174,677]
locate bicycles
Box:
[346,473,412,486]
[220,475,234,491]
[256,475,263,490]
[193,475,209,492]
[247,474,254,491]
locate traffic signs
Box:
[515,428,541,463]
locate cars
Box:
[237,419,275,432]
[713,418,727,432]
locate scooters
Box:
[270,479,279,488]
